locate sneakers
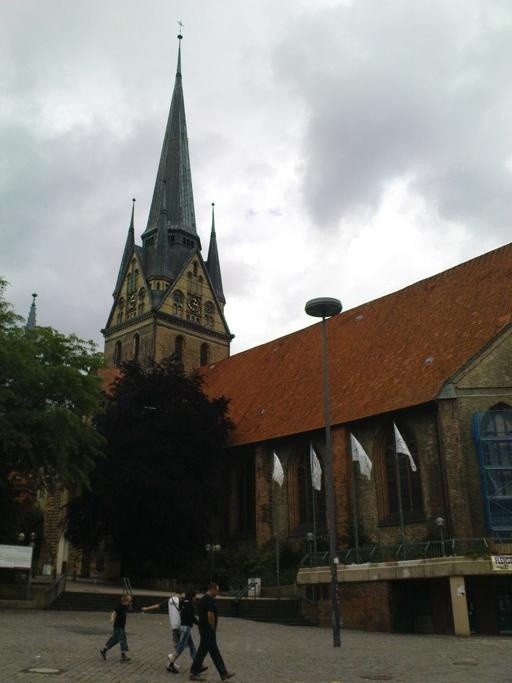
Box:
[166,653,236,681]
[100,646,131,662]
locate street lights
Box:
[304,296,344,649]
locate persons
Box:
[100,593,136,662]
[140,585,184,672]
[187,582,237,682]
[164,586,210,675]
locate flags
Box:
[348,433,375,481]
[392,424,417,473]
[270,450,285,487]
[308,446,325,493]
